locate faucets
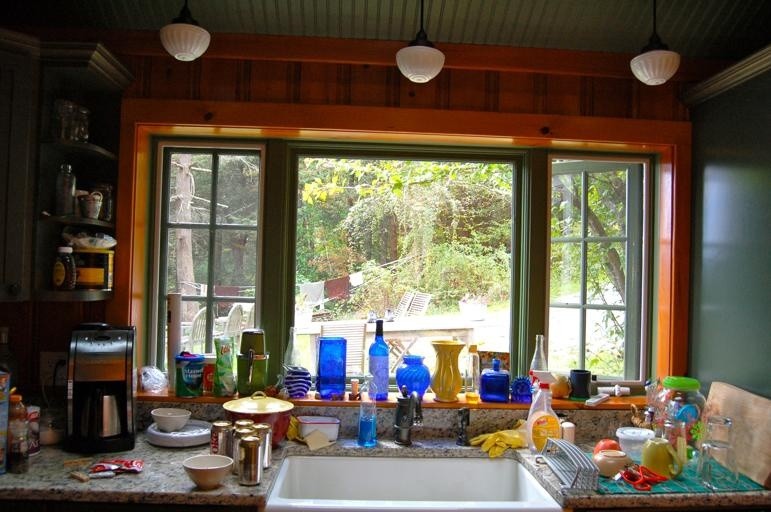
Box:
[394,387,422,445]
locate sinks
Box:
[265,455,564,512]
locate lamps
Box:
[630,1,682,87]
[158,0,214,63]
[395,1,446,85]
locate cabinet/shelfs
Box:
[39,38,137,304]
[1,26,39,306]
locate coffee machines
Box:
[62,321,139,459]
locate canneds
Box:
[210,420,272,486]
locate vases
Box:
[395,354,432,402]
[530,333,549,383]
[430,340,468,404]
[283,366,314,399]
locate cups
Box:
[664,419,689,471]
[570,369,591,401]
[694,416,742,493]
[73,189,104,220]
[240,329,267,357]
[640,436,683,482]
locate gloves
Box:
[469,425,528,458]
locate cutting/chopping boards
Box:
[697,381,771,488]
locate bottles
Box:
[50,246,77,290]
[283,325,300,384]
[562,420,575,445]
[615,383,622,398]
[479,355,511,404]
[101,184,114,221]
[369,320,390,402]
[465,344,482,403]
[589,374,600,397]
[38,407,64,445]
[54,162,74,216]
[49,96,90,145]
[528,332,548,380]
[645,378,655,424]
[8,394,31,473]
[203,353,218,392]
[653,374,707,464]
[358,376,379,448]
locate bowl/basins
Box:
[220,407,293,445]
[593,437,622,459]
[592,450,633,479]
[150,406,191,434]
[615,425,654,460]
[181,454,243,486]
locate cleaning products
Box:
[528,370,562,454]
